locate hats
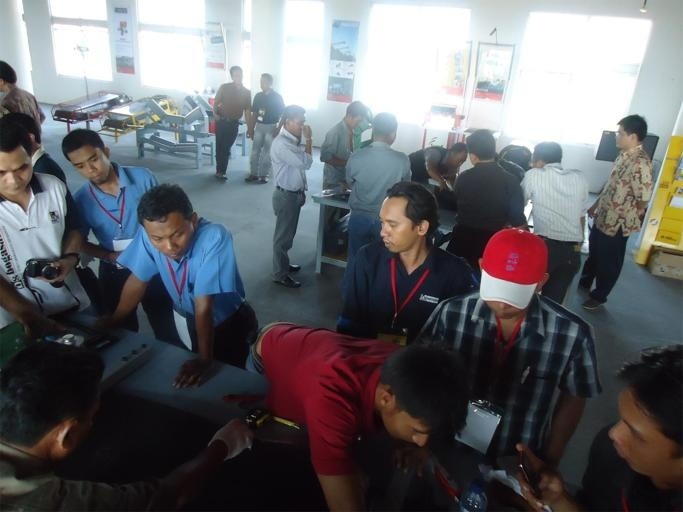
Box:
[480,227,550,310]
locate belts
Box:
[277,186,301,193]
[221,116,243,125]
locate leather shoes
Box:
[288,264,301,272]
[260,175,268,183]
[245,175,259,182]
[273,275,301,288]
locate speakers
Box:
[596,131,659,165]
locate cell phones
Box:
[518,449,543,497]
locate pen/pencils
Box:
[272,417,300,429]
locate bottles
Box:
[456,481,488,512]
[61,333,76,347]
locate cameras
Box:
[26,259,58,280]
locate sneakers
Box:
[583,297,607,310]
[215,169,227,180]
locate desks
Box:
[310,178,532,275]
